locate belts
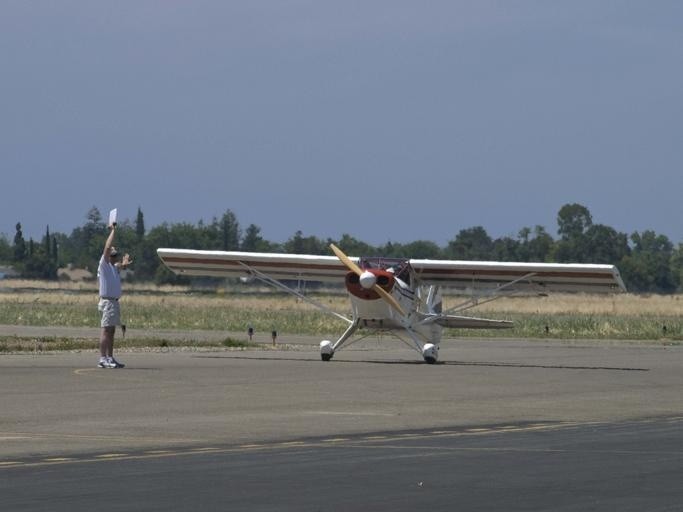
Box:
[100,296,120,300]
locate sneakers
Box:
[97,357,124,369]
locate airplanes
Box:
[156,241,629,364]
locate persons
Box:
[96,225,133,370]
